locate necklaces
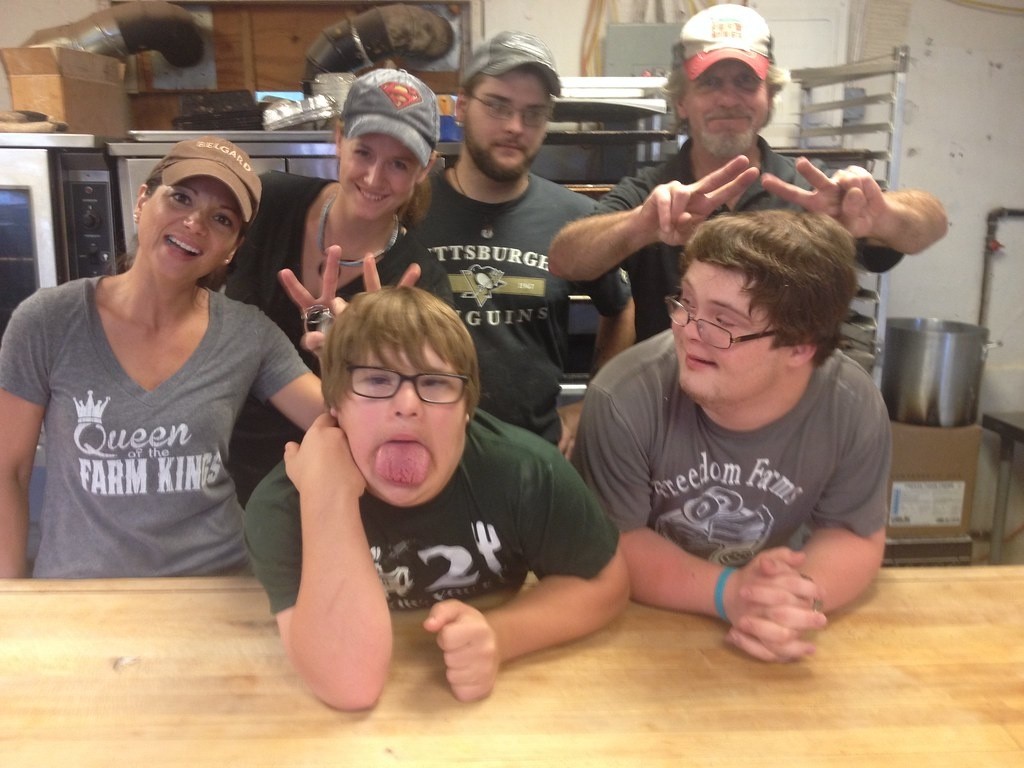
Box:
[318,195,399,279]
[454,170,522,239]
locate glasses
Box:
[345,363,468,404]
[665,295,783,349]
[468,93,551,127]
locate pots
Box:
[880,315,1003,428]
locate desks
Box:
[0,559,1024,768]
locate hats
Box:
[459,30,562,97]
[672,4,774,81]
[145,136,262,231]
[339,68,441,170]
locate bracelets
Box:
[715,566,734,621]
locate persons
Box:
[570,211,892,663]
[548,5,949,348]
[241,284,629,711]
[205,68,454,503]
[1,138,348,576]
[411,31,636,460]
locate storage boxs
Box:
[888,420,985,538]
[0,46,135,135]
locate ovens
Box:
[0,147,121,346]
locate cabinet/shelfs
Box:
[720,43,907,389]
